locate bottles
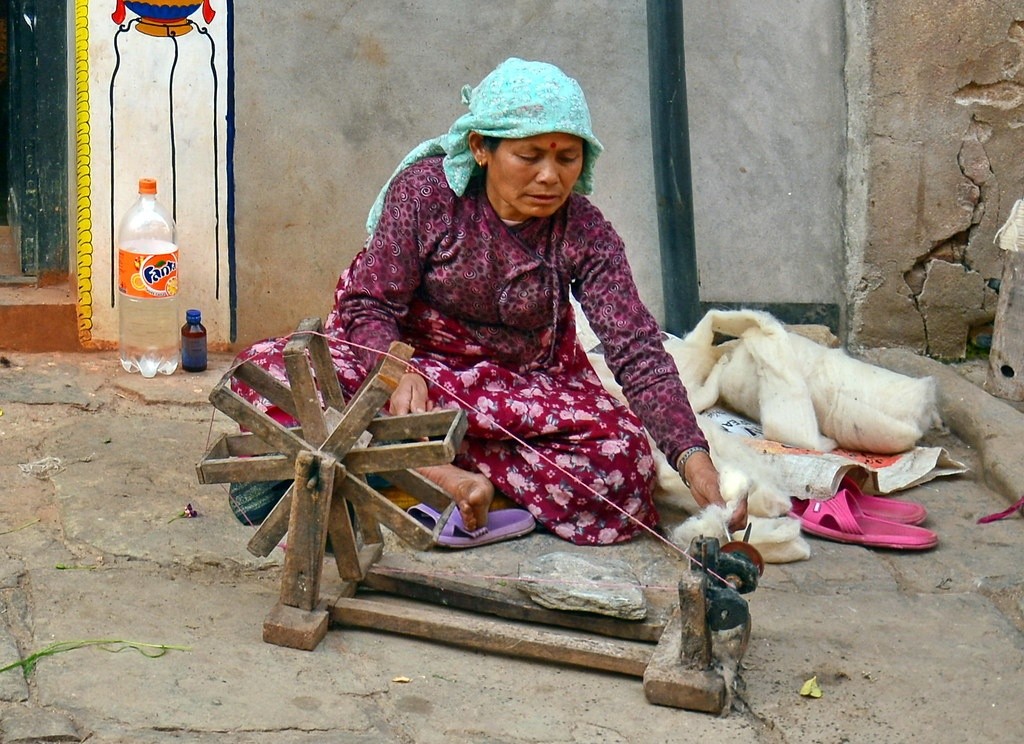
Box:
[117,177,181,377]
[181,309,208,372]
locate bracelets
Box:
[678,446,710,489]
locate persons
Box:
[228,57,748,534]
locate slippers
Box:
[786,489,938,549]
[837,477,928,526]
[408,504,534,549]
